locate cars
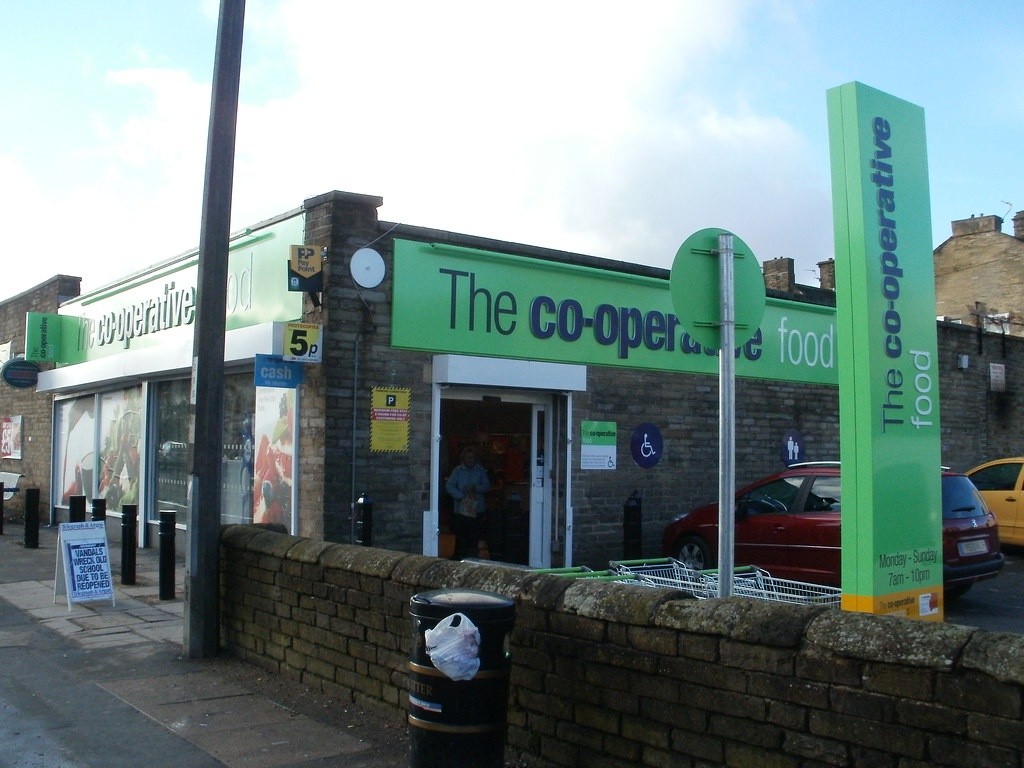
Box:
[962,459,1023,544]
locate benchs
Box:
[0,472,25,501]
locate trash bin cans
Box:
[407,587,518,768]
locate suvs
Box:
[654,458,1002,603]
[157,442,188,469]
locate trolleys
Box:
[529,555,843,610]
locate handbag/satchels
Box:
[424,612,480,681]
[459,487,480,517]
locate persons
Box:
[446,447,491,559]
